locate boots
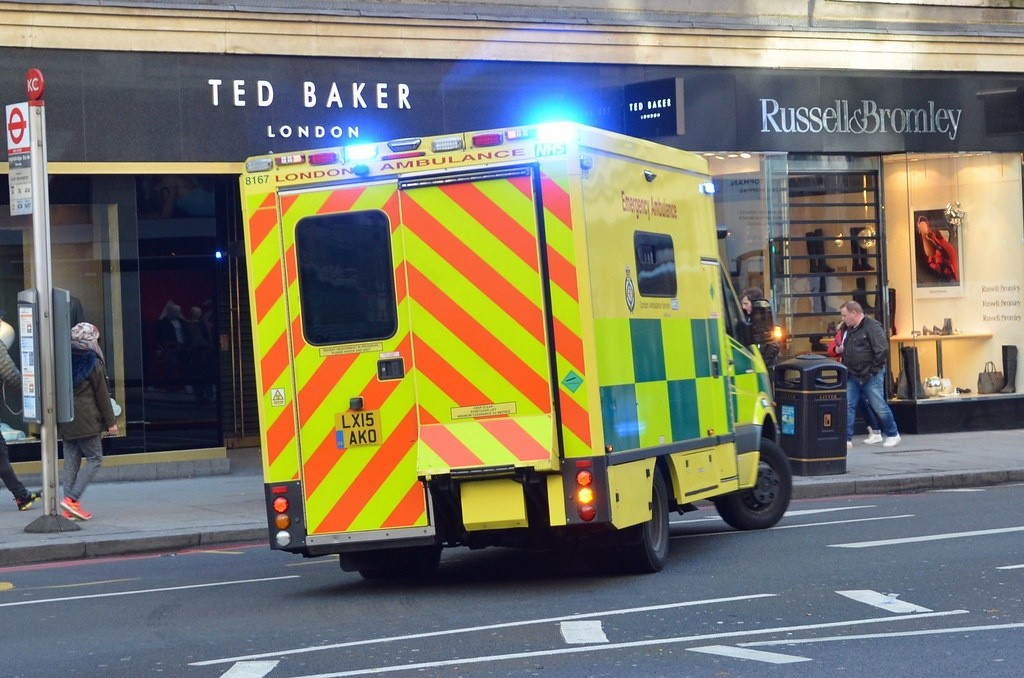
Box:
[849,227,876,271]
[901,346,930,399]
[851,278,874,310]
[810,278,838,312]
[1002,345,1018,394]
[809,336,828,352]
[942,318,952,335]
[938,379,953,396]
[806,229,836,273]
[887,289,897,335]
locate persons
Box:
[0,318,43,510]
[151,300,216,393]
[58,322,114,522]
[735,290,779,366]
[828,301,900,440]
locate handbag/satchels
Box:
[977,362,1004,395]
[897,359,910,398]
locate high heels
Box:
[923,325,941,336]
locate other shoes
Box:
[957,388,971,394]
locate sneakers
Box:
[862,426,882,444]
[58,497,92,520]
[13,488,44,510]
[882,434,901,446]
[847,441,853,449]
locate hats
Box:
[70,322,99,342]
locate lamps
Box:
[945,203,966,221]
[857,227,877,249]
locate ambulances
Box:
[239,120,794,590]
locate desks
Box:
[819,334,995,378]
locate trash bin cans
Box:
[772,352,849,478]
